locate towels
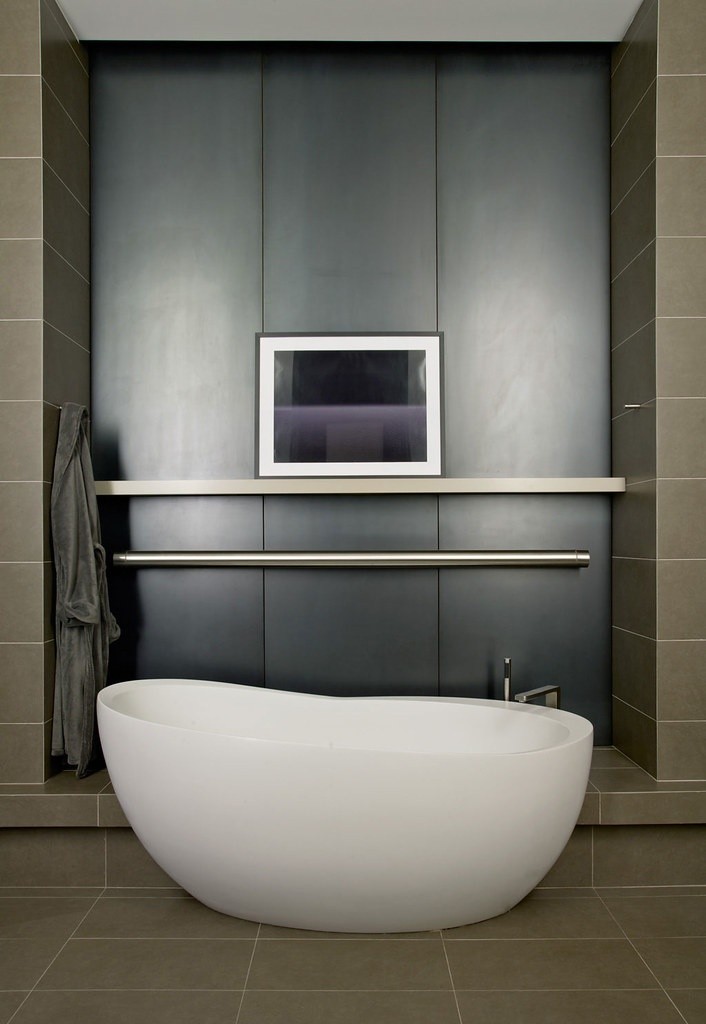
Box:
[50,401,123,780]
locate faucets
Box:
[514,684,563,710]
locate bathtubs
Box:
[95,677,595,935]
